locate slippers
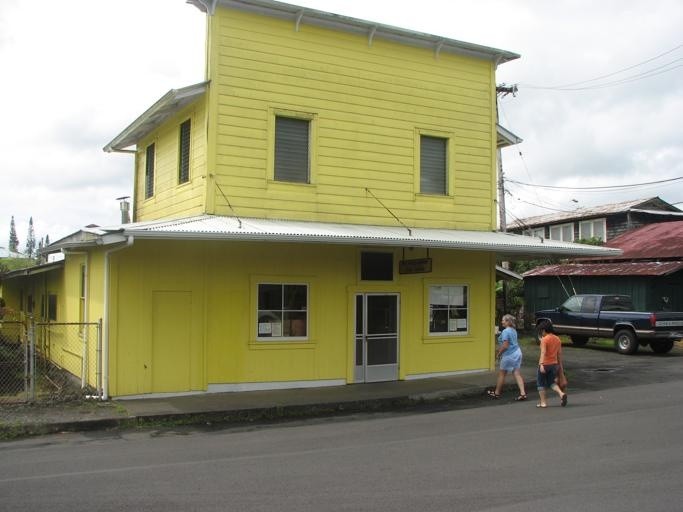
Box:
[561,394,567,407]
[536,402,548,408]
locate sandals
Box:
[488,391,501,399]
[515,393,527,400]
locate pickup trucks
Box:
[529,294,683,355]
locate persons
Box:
[536,319,567,408]
[488,314,528,401]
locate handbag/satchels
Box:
[557,368,567,391]
